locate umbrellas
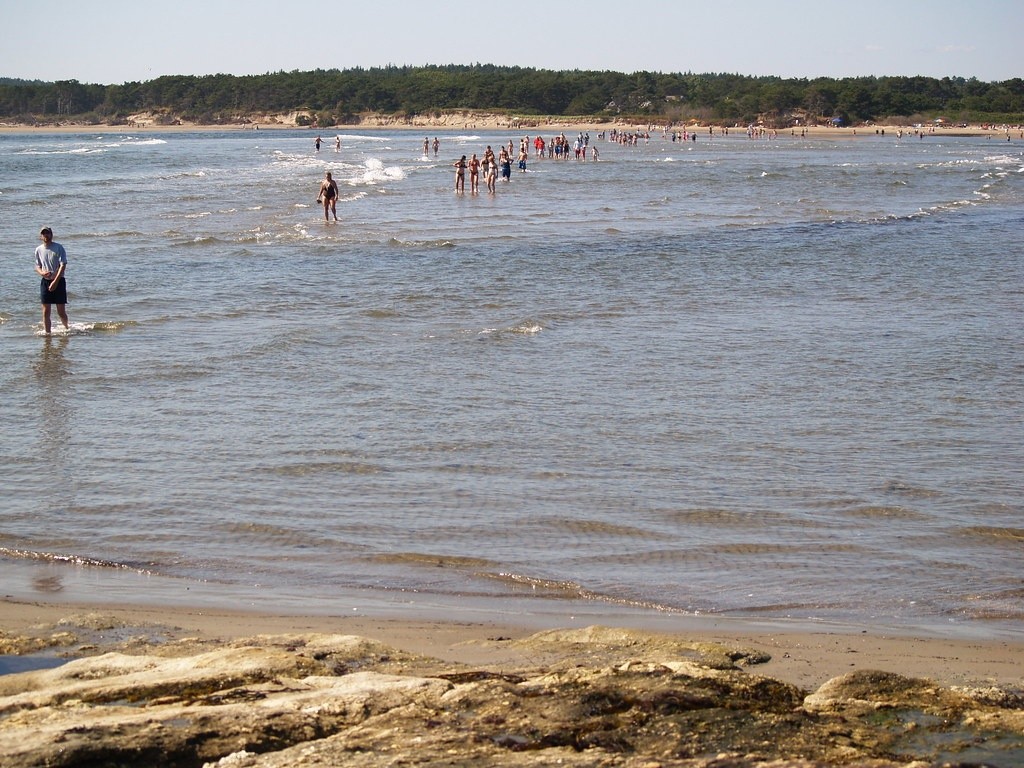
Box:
[934,118,945,123]
[832,118,842,122]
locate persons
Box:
[423,137,429,155]
[432,137,439,154]
[495,116,594,130]
[317,173,339,221]
[314,136,323,151]
[590,146,599,161]
[453,135,529,195]
[34,227,69,333]
[335,137,341,152]
[597,117,1024,146]
[534,132,590,160]
